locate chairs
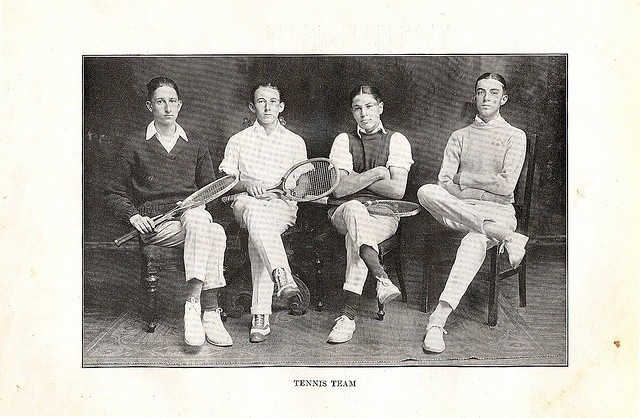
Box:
[421,131,539,326]
[138,233,227,333]
[222,207,313,318]
[315,217,414,320]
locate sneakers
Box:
[202,307,233,347]
[375,275,401,305]
[499,232,530,270]
[325,315,356,344]
[183,297,205,346]
[422,323,448,354]
[273,267,301,302]
[249,314,271,342]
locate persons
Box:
[416,71,529,355]
[102,77,234,347]
[218,82,316,342]
[325,84,416,345]
